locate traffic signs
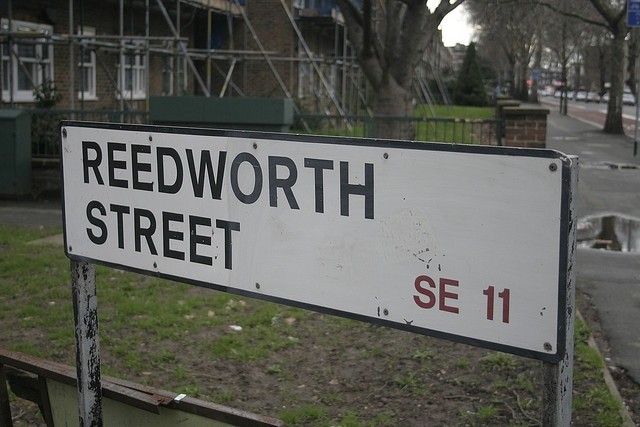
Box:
[58,119,571,365]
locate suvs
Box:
[623,90,636,105]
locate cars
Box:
[585,92,600,103]
[554,90,587,101]
